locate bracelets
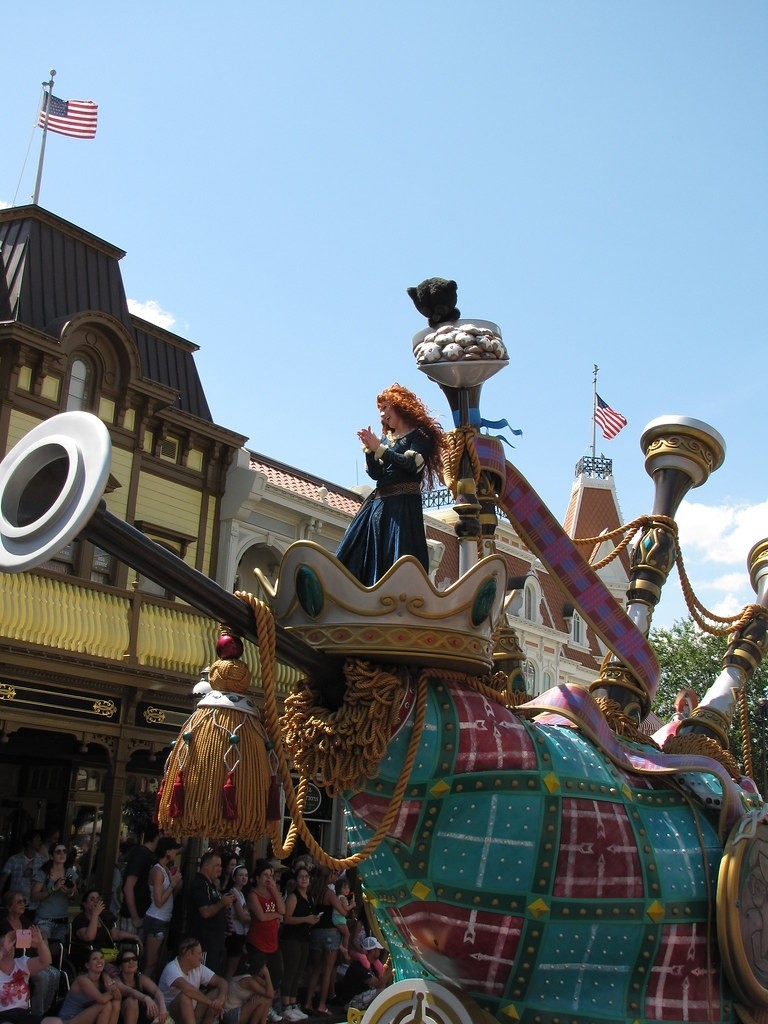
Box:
[48,885,55,895]
[142,995,146,1000]
[169,884,174,888]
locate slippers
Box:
[317,1008,333,1017]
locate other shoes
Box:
[270,1009,282,1022]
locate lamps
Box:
[318,484,328,505]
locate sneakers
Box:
[281,1005,302,1022]
[292,1004,308,1019]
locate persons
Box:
[0,823,393,1024]
[337,384,447,588]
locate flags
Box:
[593,394,627,439]
[39,89,98,139]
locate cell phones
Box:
[66,875,74,887]
[316,912,324,918]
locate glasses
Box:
[298,875,310,879]
[12,899,26,904]
[54,849,68,855]
[122,956,137,963]
[89,897,103,902]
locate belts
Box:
[43,917,68,925]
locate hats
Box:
[157,837,183,851]
[360,937,384,950]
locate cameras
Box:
[16,929,33,941]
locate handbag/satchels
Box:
[100,947,119,961]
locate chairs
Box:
[23,922,140,1014]
